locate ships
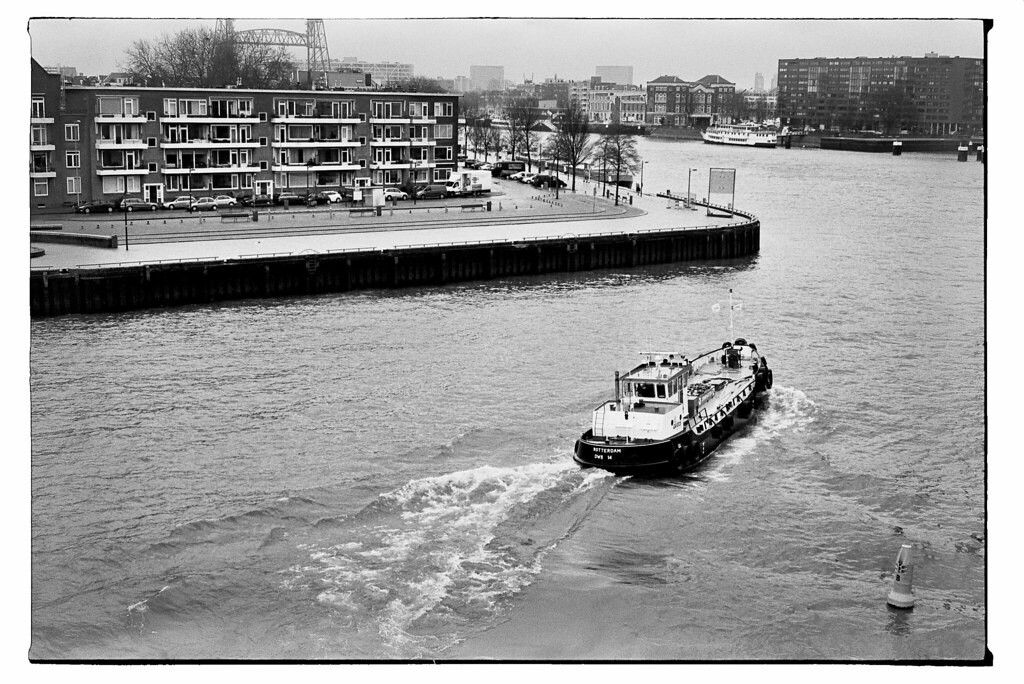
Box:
[700,116,777,148]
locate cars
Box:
[301,193,331,208]
[383,187,408,200]
[275,191,307,207]
[321,190,342,203]
[185,197,219,212]
[241,194,276,207]
[213,195,237,207]
[463,155,569,187]
[161,196,195,211]
[74,199,116,215]
[118,198,158,213]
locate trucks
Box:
[446,170,492,200]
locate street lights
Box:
[593,151,697,209]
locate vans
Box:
[413,184,447,198]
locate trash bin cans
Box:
[377,206,381,215]
[393,196,397,205]
[252,210,259,221]
[111,234,118,248]
[487,200,492,211]
[284,199,289,210]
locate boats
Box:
[570,338,772,477]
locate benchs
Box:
[609,192,630,204]
[461,204,485,212]
[348,208,376,217]
[220,213,250,223]
